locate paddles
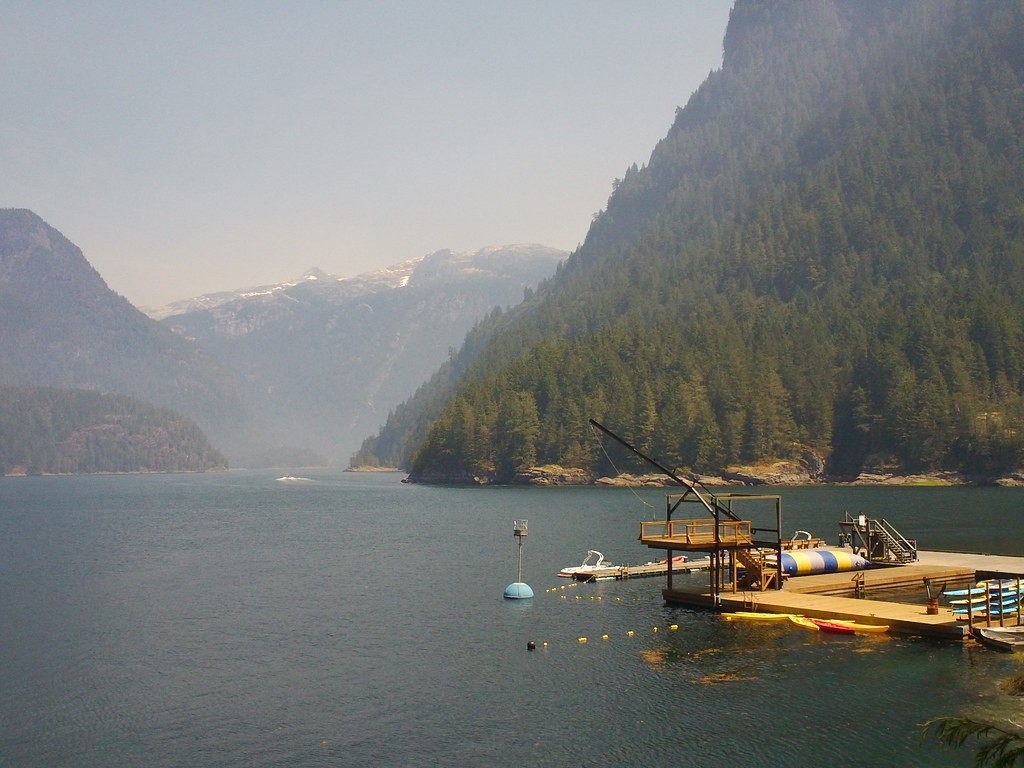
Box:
[922,576,933,598]
[936,582,947,598]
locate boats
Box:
[788,616,820,633]
[804,617,856,636]
[974,627,1024,654]
[643,555,689,566]
[830,618,890,638]
[720,610,805,623]
[557,550,625,580]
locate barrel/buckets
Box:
[927,599,938,615]
